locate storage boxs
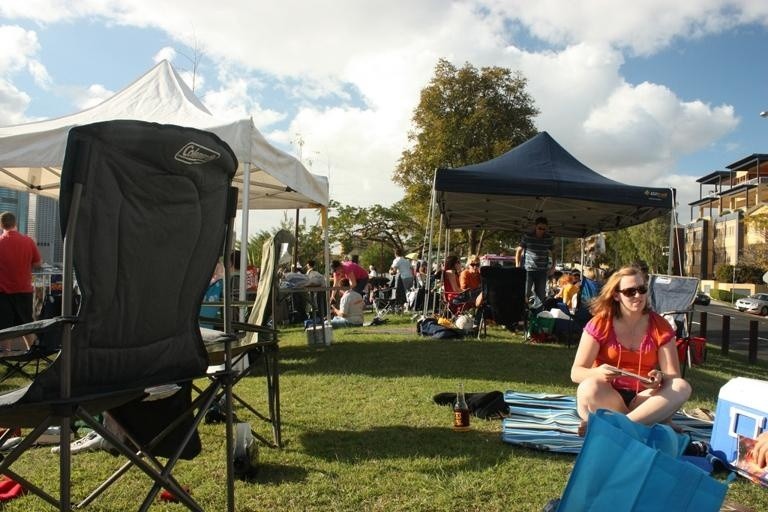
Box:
[709,375,768,467]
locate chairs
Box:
[439,270,475,322]
[475,267,530,339]
[369,277,399,319]
[0,119,240,512]
[635,274,700,372]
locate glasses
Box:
[615,286,647,297]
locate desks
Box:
[224,283,350,345]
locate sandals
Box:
[487,404,512,419]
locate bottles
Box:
[453,382,470,433]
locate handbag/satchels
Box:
[555,409,737,512]
[676,337,707,364]
[433,391,504,419]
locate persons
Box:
[753,430,768,467]
[568,265,692,437]
[305,213,598,331]
[35,274,80,354]
[199,246,304,329]
[1,208,46,353]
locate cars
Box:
[734,293,767,315]
[694,288,710,305]
